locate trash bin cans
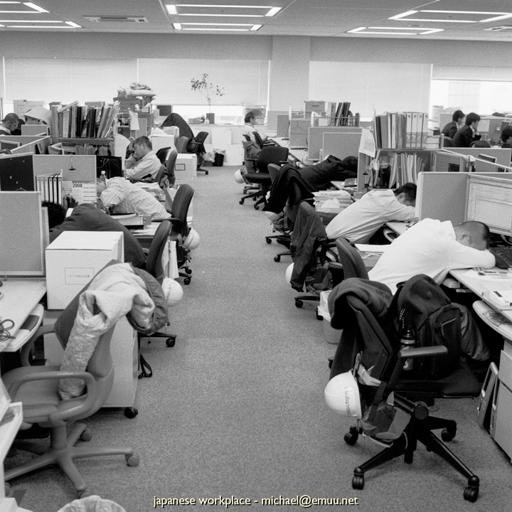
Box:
[212,148,226,166]
[317,290,344,343]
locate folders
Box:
[35,171,64,205]
[404,112,429,149]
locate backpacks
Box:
[384,273,462,381]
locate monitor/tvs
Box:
[0,153,34,191]
[478,153,497,163]
[447,162,465,172]
[466,177,512,235]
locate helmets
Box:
[182,226,200,250]
[285,262,306,293]
[161,276,184,305]
[233,168,247,184]
[324,368,362,421]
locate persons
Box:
[442,110,465,138]
[367,217,497,383]
[97,175,171,224]
[471,140,490,148]
[122,136,169,188]
[298,154,358,198]
[241,111,268,148]
[316,183,417,263]
[502,126,512,162]
[449,112,481,146]
[0,113,19,136]
[42,199,146,269]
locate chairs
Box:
[237,102,512,505]
[1,94,214,511]
